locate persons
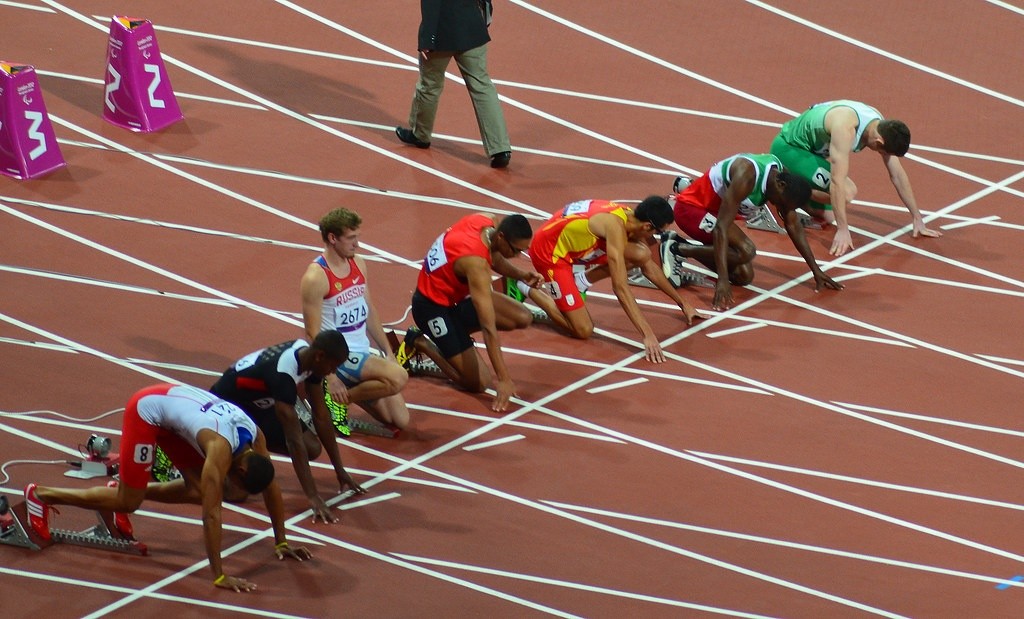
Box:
[299,207,411,438]
[738,99,944,257]
[395,213,545,411]
[24,383,314,593]
[504,194,711,364]
[152,329,369,526]
[396,0,512,168]
[658,152,846,312]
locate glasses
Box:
[507,240,523,254]
[649,219,665,235]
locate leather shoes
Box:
[395,126,431,148]
[491,152,511,169]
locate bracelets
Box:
[273,540,289,551]
[214,574,225,585]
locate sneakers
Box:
[506,277,526,303]
[659,239,684,287]
[396,325,424,375]
[660,229,678,243]
[325,393,351,436]
[573,272,588,304]
[24,481,60,541]
[107,480,133,535]
[151,446,172,483]
